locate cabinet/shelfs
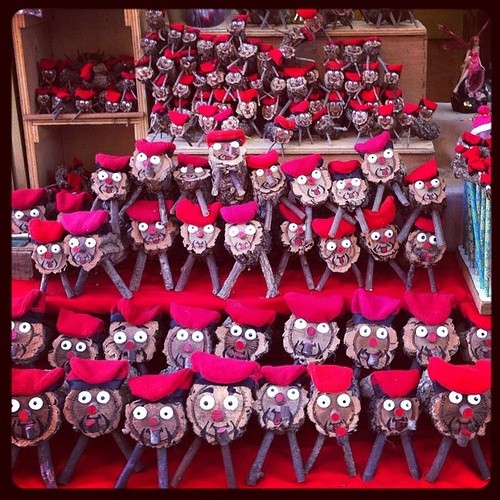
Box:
[6,8,490,490]
